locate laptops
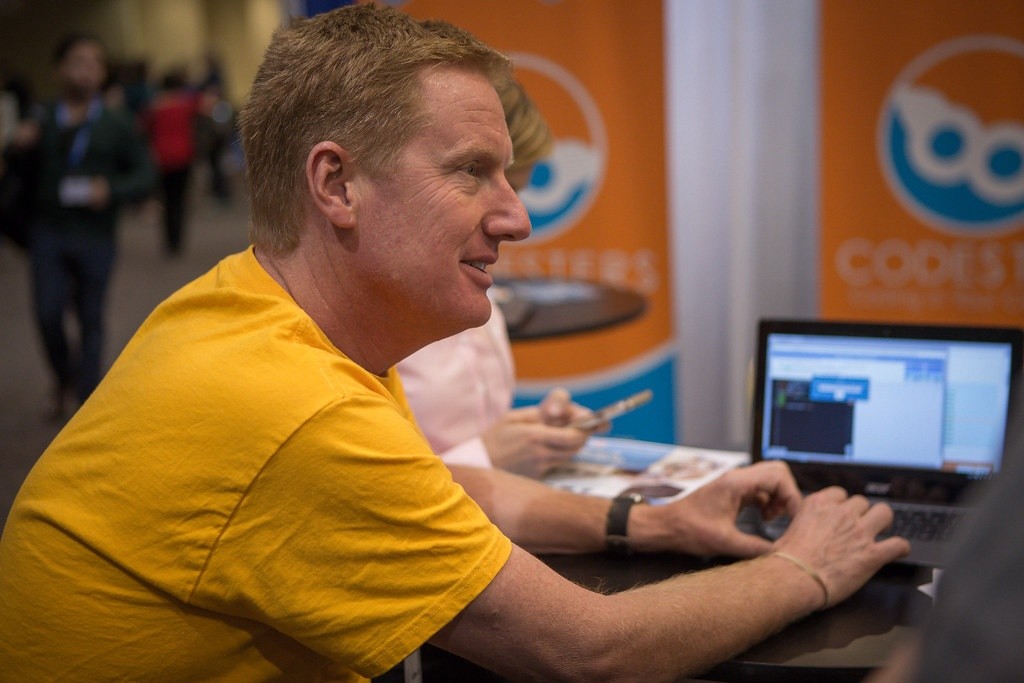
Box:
[731,319,1024,566]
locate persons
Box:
[392,73,603,485]
[0,31,233,415]
[0,1,911,683]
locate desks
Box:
[496,277,645,341]
[404,548,950,682]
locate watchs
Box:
[605,491,648,557]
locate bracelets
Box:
[772,551,831,604]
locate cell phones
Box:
[566,389,653,431]
